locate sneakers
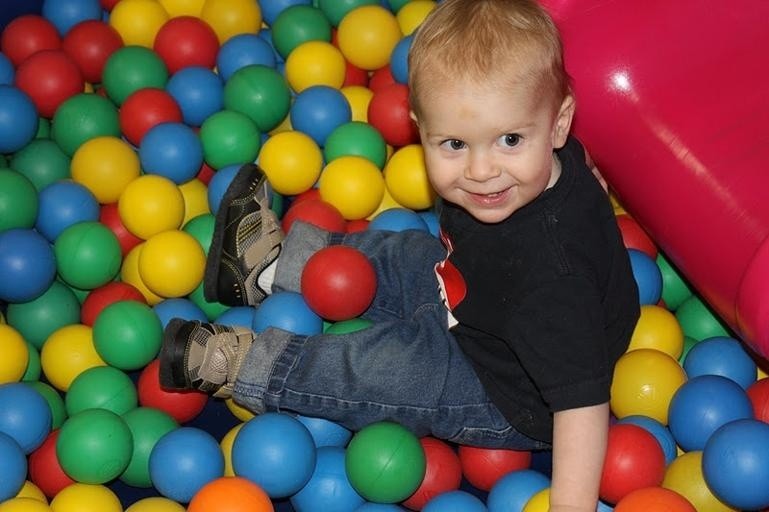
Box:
[159,318,252,399]
[204,163,285,307]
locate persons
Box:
[158,1,642,512]
